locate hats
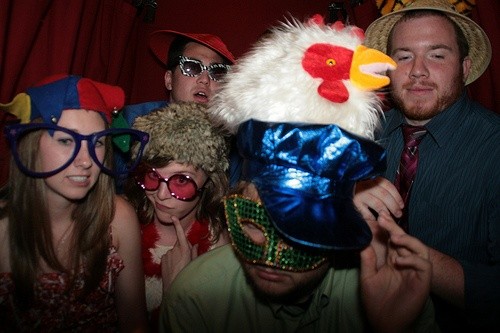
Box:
[363,1,492,88]
[146,27,238,66]
[128,104,229,173]
[237,118,390,253]
[0,74,126,138]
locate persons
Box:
[365,0,500,333]
[112,21,439,333]
[1,73,149,333]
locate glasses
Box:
[178,56,233,82]
[6,121,149,179]
[135,167,210,201]
